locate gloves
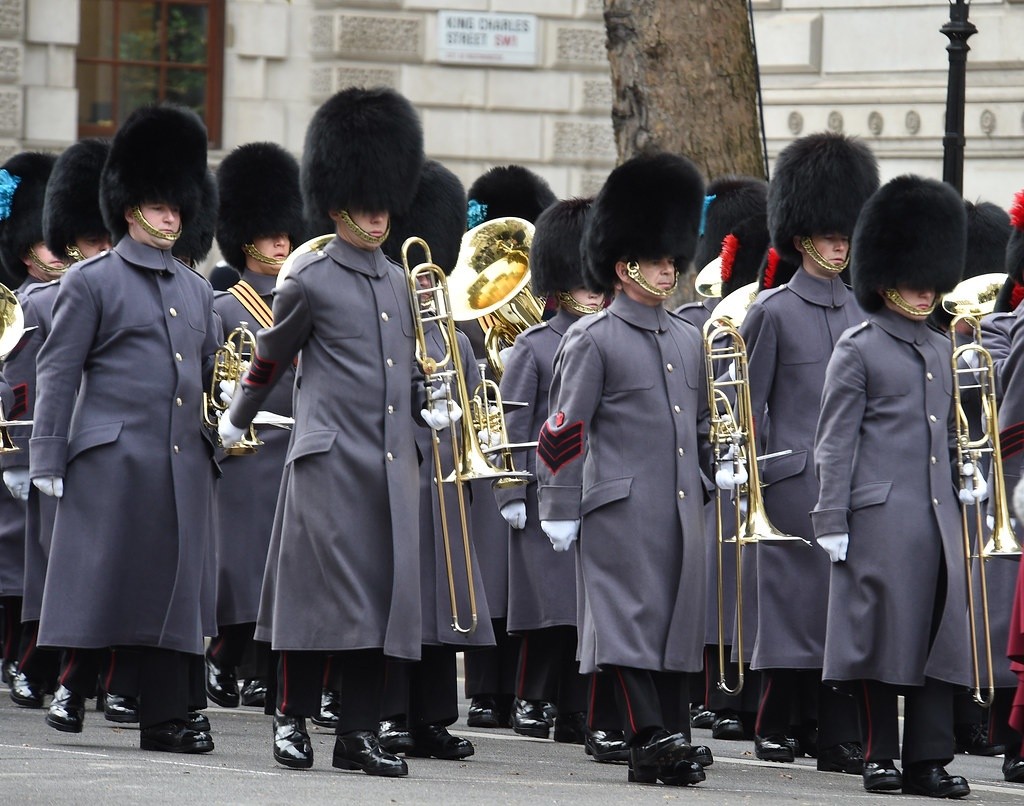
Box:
[500,498,528,531]
[714,452,749,491]
[478,405,504,463]
[539,517,581,553]
[2,466,31,502]
[216,408,248,450]
[219,378,239,407]
[32,476,64,498]
[957,462,989,507]
[986,514,1016,530]
[816,533,850,563]
[418,382,463,434]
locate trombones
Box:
[949,312,1024,709]
[700,315,814,697]
[399,234,536,637]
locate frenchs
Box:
[0,211,1012,387]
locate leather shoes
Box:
[268,707,315,767]
[139,722,216,755]
[206,647,269,707]
[682,738,714,767]
[956,723,1008,758]
[815,741,865,775]
[863,757,903,791]
[95,684,140,725]
[310,688,342,727]
[754,732,820,763]
[686,703,717,727]
[374,714,477,760]
[897,761,966,799]
[43,682,87,734]
[469,693,558,738]
[182,707,214,733]
[552,710,589,743]
[626,731,708,790]
[711,711,756,741]
[583,728,628,762]
[999,751,1024,781]
[331,728,411,780]
[0,640,45,708]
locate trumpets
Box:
[201,321,267,457]
[460,363,531,491]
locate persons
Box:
[2,88,1023,799]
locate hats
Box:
[580,152,706,299]
[299,85,424,237]
[695,131,883,300]
[849,174,1024,317]
[210,140,306,291]
[383,164,597,297]
[0,101,220,293]
